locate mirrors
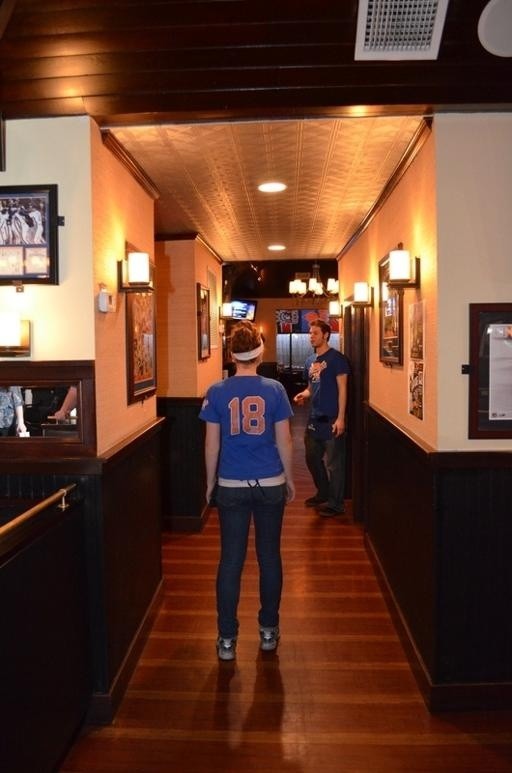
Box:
[0,386,78,438]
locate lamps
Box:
[328,301,343,319]
[118,252,155,293]
[352,282,374,311]
[219,303,234,324]
[384,250,420,288]
[289,259,339,305]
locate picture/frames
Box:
[0,185,59,286]
[469,303,512,440]
[126,240,157,405]
[197,283,210,360]
[378,242,403,366]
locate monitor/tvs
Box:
[232,299,257,321]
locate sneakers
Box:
[304,496,328,506]
[313,501,345,517]
[215,635,238,661]
[259,626,281,651]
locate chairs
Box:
[288,369,308,405]
[259,362,280,380]
[41,416,78,438]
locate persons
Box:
[31,386,78,436]
[198,319,296,661]
[293,319,351,517]
[386,341,393,356]
[0,205,47,244]
[0,386,27,436]
